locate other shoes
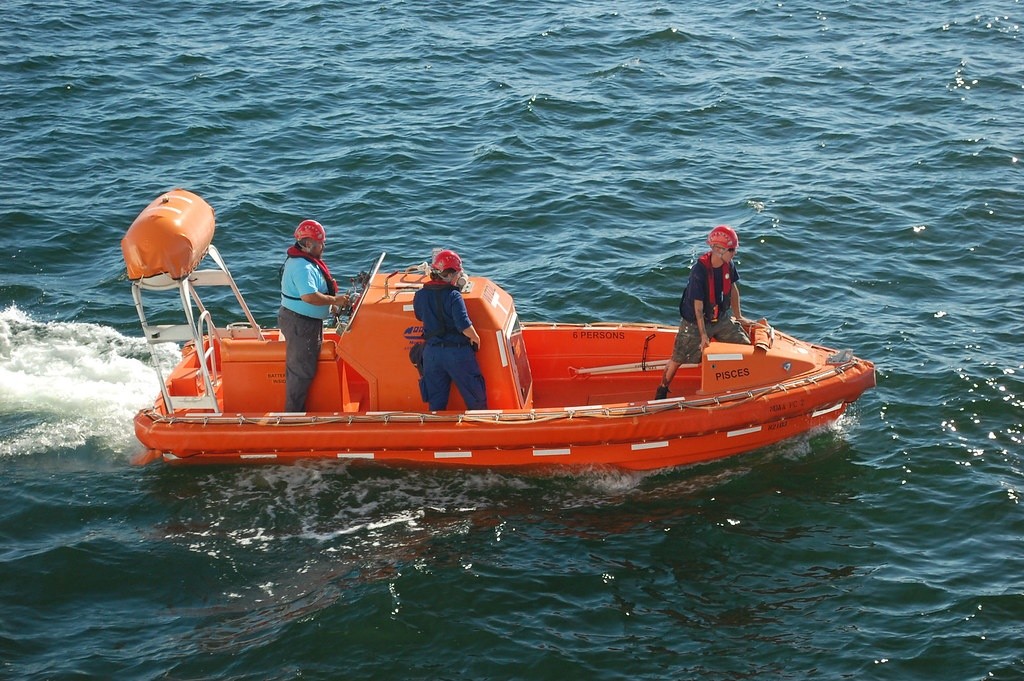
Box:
[655,384,669,400]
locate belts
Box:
[428,340,471,348]
[282,306,322,321]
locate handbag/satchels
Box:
[409,342,426,377]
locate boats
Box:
[120,186,878,468]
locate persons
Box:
[655,224,751,400]
[413,250,488,410]
[277,219,350,412]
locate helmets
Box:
[432,249,464,272]
[705,226,740,248]
[293,219,327,243]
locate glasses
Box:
[715,245,735,253]
